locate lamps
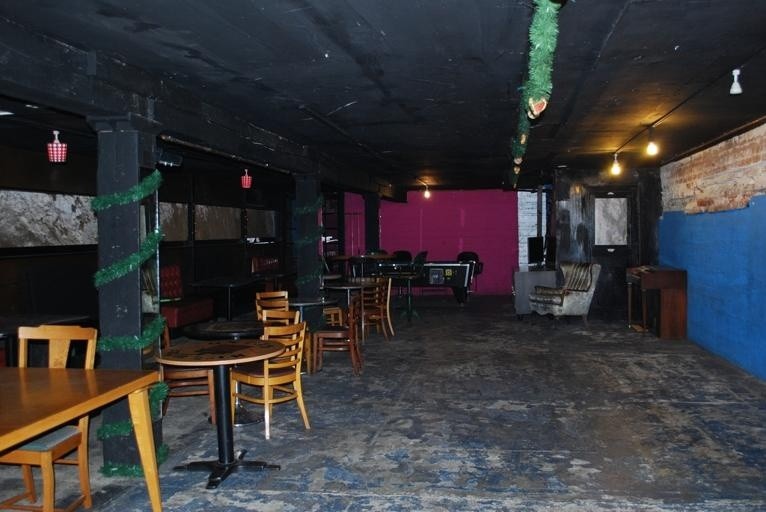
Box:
[730,69,743,94]
[241,169,251,188]
[611,153,621,175]
[647,127,659,155]
[48,130,68,162]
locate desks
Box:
[156,339,286,488]
[384,260,474,310]
[288,297,339,322]
[0,367,162,512]
[192,275,261,320]
[627,265,687,339]
[333,254,392,261]
[210,320,286,423]
[1,313,90,367]
[323,274,342,299]
[327,284,378,348]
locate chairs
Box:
[257,289,288,325]
[456,251,483,291]
[324,306,342,326]
[348,257,377,283]
[396,251,429,321]
[364,277,395,336]
[0,325,97,512]
[355,284,390,342]
[311,296,361,373]
[391,250,411,261]
[236,310,299,405]
[230,322,311,440]
[158,323,216,425]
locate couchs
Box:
[251,255,295,294]
[160,264,212,338]
[529,263,602,328]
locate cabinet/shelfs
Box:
[512,267,556,320]
